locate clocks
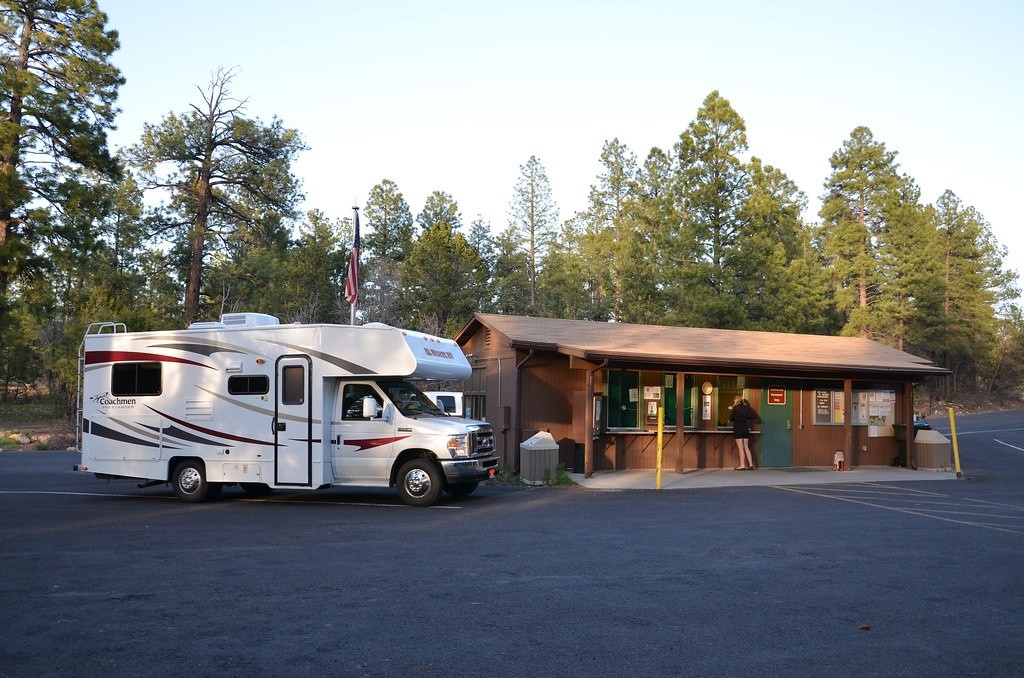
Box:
[702,382,712,395]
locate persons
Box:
[730,394,756,470]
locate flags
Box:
[342,212,360,305]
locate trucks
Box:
[73,314,501,505]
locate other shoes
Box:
[749,466,753,470]
[734,467,745,471]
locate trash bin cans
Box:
[519,430,560,486]
[914,429,953,472]
